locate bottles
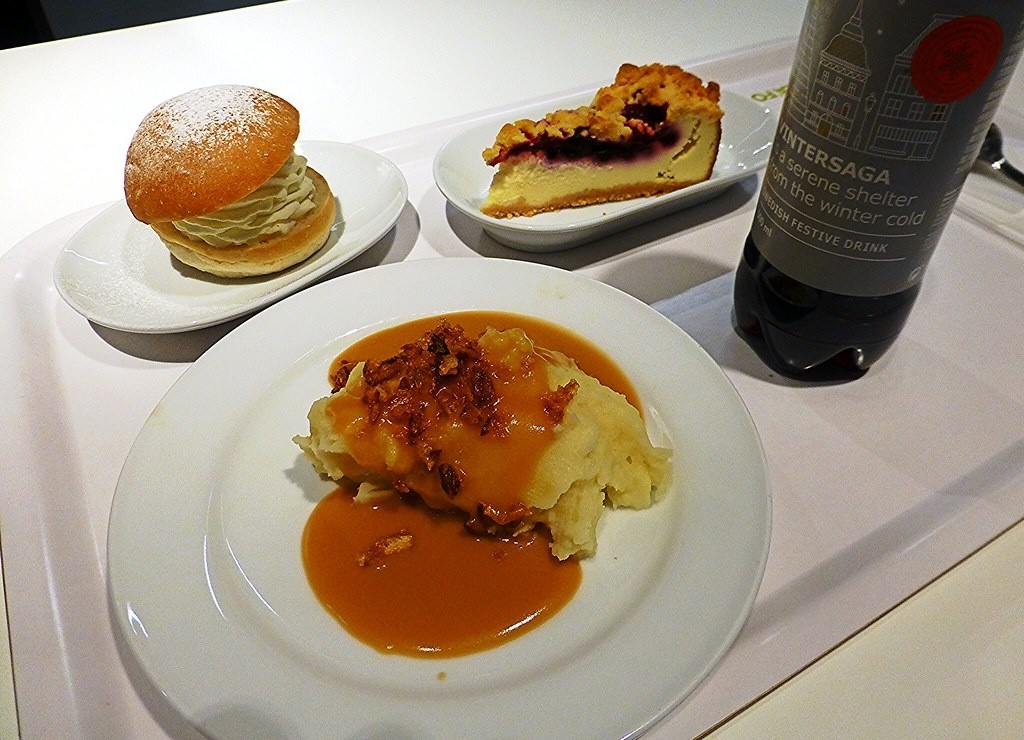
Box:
[726,0,1024,380]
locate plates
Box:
[52,142,406,334]
[434,90,779,254]
[107,257,773,740]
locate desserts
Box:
[480,62,724,217]
[291,322,673,563]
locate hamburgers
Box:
[125,85,338,278]
